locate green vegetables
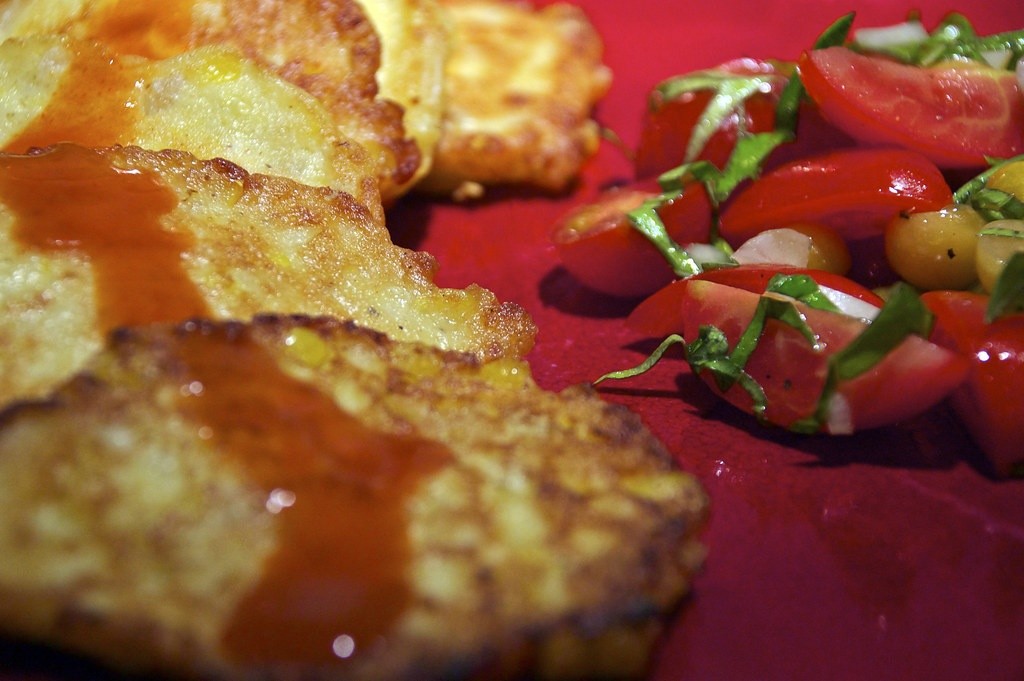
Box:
[588,11,1024,427]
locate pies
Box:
[0,0,714,681]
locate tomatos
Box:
[553,47,1024,482]
